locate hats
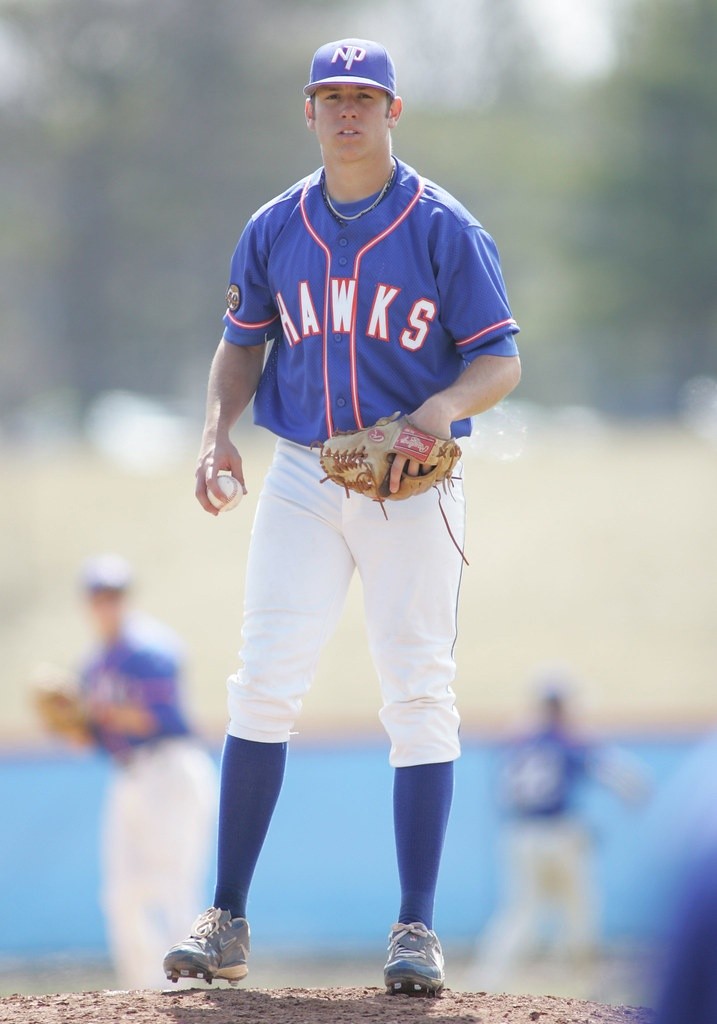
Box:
[303,38,395,102]
[80,557,134,591]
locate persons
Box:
[48,553,222,991]
[465,678,646,987]
[162,36,525,998]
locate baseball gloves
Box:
[319,411,464,503]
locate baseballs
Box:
[204,475,245,514]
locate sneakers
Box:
[382,920,446,997]
[163,908,250,984]
